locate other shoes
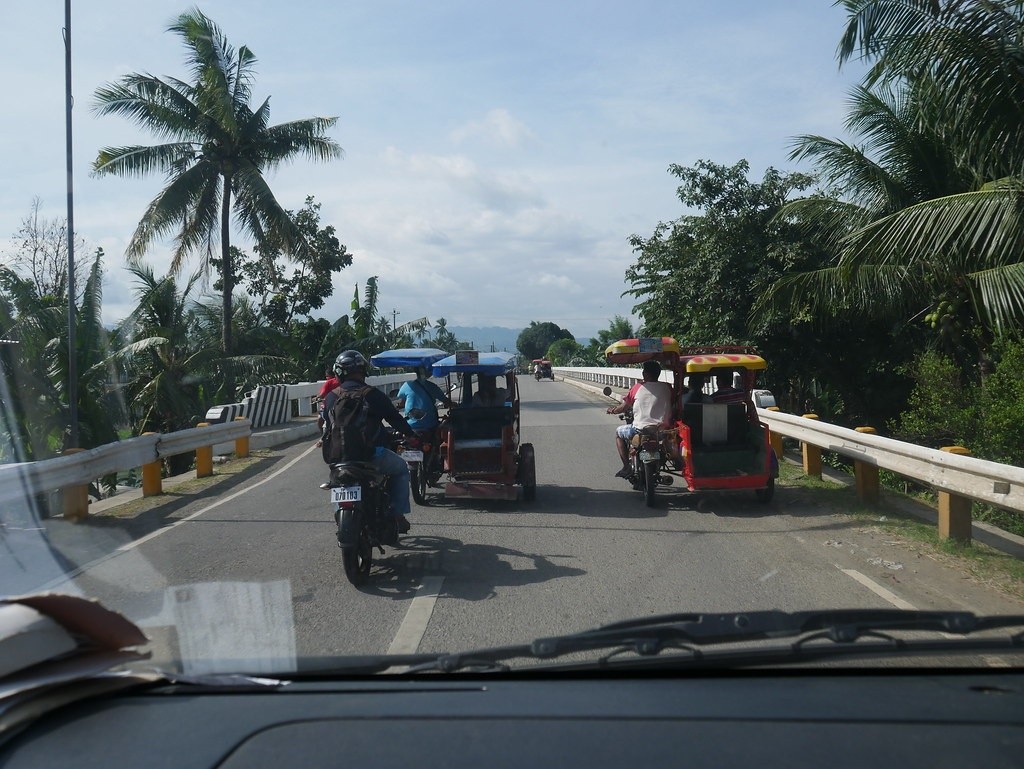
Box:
[434,464,441,469]
[615,471,633,477]
[396,513,410,530]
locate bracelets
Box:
[612,407,617,414]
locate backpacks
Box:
[322,385,376,464]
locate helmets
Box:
[414,362,433,377]
[333,350,369,379]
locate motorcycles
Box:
[389,382,457,505]
[319,408,430,586]
[534,371,543,382]
[603,386,678,507]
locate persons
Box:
[471,376,510,407]
[311,368,339,434]
[676,375,714,409]
[528,363,533,371]
[607,361,675,477]
[398,366,454,468]
[324,350,415,532]
[534,362,541,373]
[710,370,760,424]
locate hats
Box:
[529,363,532,365]
